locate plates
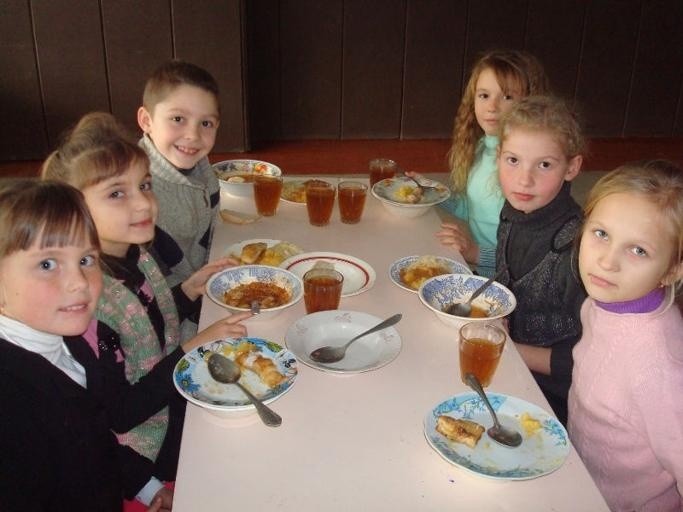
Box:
[280,179,305,207]
[285,310,403,375]
[372,177,453,207]
[423,394,569,478]
[278,252,377,298]
[389,253,473,294]
[222,239,300,266]
[174,338,298,412]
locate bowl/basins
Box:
[209,159,283,198]
[417,274,518,328]
[372,180,436,220]
[203,264,304,318]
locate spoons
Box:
[446,263,512,318]
[465,372,523,448]
[309,311,404,365]
[206,354,284,429]
[402,169,438,196]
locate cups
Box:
[306,179,367,228]
[368,157,397,188]
[254,175,282,216]
[303,268,343,314]
[458,322,508,389]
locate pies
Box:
[240,241,267,265]
[436,415,486,448]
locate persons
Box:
[566,159,683,512]
[1,176,177,512]
[494,94,589,431]
[137,61,221,345]
[405,50,551,279]
[40,111,255,477]
